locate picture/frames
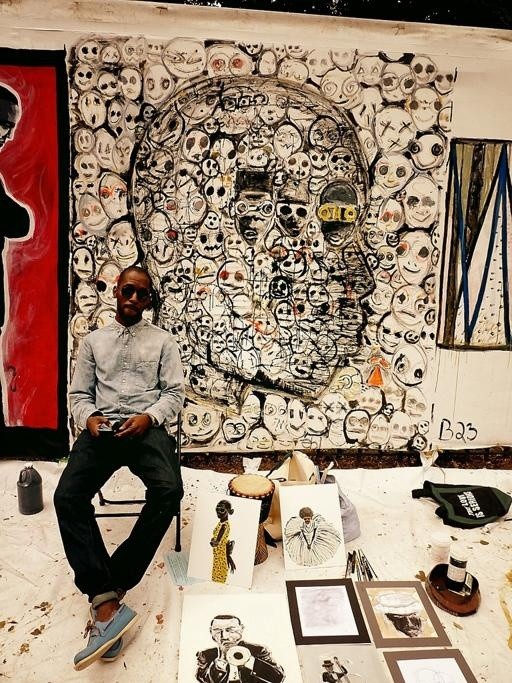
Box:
[285,579,478,683]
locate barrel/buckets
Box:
[16,462,44,515]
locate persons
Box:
[208,498,239,581]
[282,504,342,566]
[373,592,427,638]
[194,613,285,683]
[0,80,37,424]
[53,266,188,673]
[320,659,349,682]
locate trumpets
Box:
[225,640,252,665]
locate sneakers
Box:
[72,603,141,673]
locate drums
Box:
[228,472,275,565]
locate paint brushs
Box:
[345,548,379,582]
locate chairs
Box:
[94,409,181,552]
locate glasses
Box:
[118,283,149,303]
